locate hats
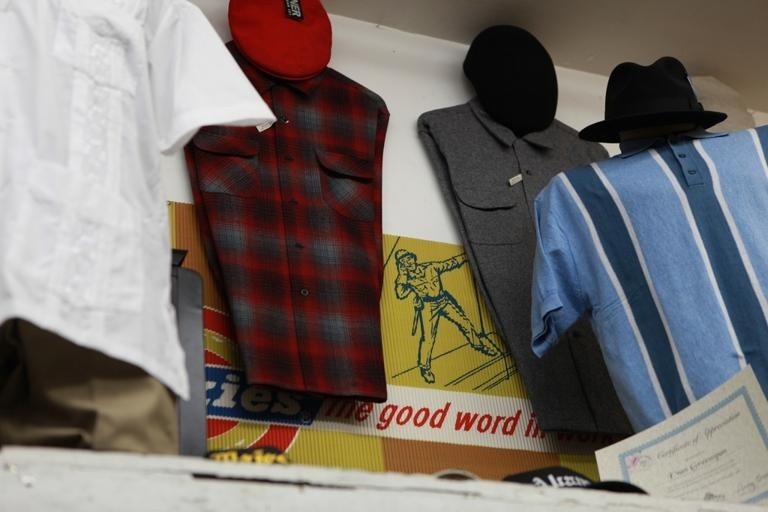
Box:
[227,1,335,84]
[501,464,650,495]
[462,23,561,136]
[576,55,730,143]
[193,445,305,484]
